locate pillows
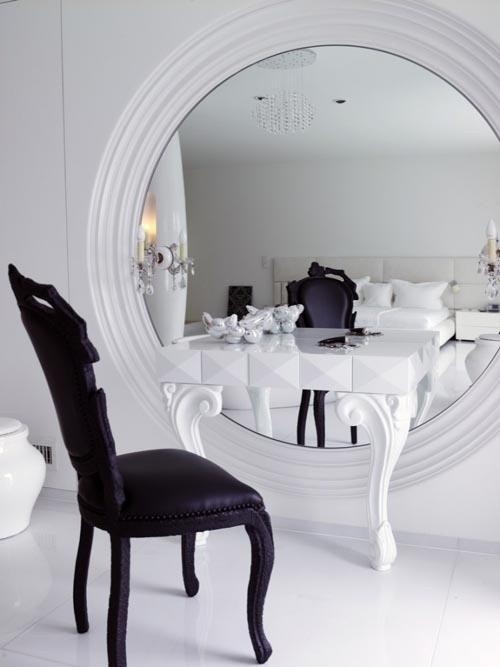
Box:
[355,275,447,312]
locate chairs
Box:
[284,262,364,449]
[6,264,277,667]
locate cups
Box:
[201,303,305,343]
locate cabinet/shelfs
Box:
[455,310,500,342]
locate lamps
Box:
[128,227,166,297]
[163,229,196,294]
[477,217,500,302]
[252,50,317,135]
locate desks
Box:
[157,342,423,576]
[244,325,437,438]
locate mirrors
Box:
[85,4,500,503]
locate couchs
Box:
[352,283,456,346]
